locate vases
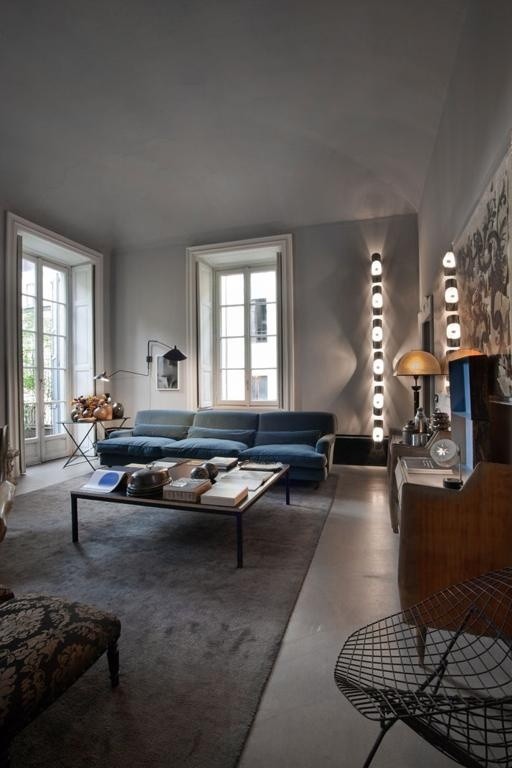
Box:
[71,406,81,422]
[94,405,108,420]
[111,403,124,418]
[83,407,93,417]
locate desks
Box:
[56,416,130,471]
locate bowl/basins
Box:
[126,469,173,496]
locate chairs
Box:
[1,479,16,545]
[334,569,512,768]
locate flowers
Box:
[98,394,111,407]
[85,396,99,408]
[71,396,86,406]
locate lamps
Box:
[372,253,383,463]
[395,350,442,416]
[443,248,461,399]
[92,340,186,382]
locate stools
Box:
[0,582,122,759]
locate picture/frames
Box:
[155,355,180,391]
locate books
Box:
[206,457,238,471]
[163,478,248,506]
[81,469,127,494]
[403,458,453,474]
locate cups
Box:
[412,432,428,448]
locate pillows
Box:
[189,426,255,440]
[256,430,320,445]
[132,424,188,439]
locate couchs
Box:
[95,410,338,490]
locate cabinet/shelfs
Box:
[388,401,512,667]
